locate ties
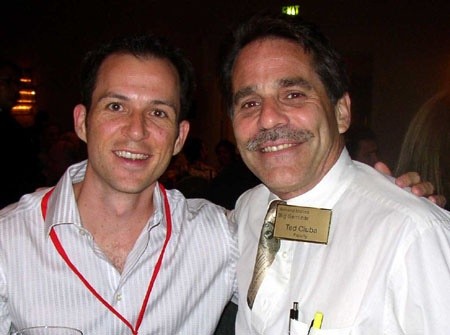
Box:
[246,200,288,310]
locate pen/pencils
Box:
[288,302,299,335]
[307,312,324,335]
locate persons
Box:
[347,128,377,168]
[396,89,450,212]
[1,59,88,210]
[220,17,450,335]
[0,38,446,335]
[158,137,261,210]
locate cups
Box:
[13,326,83,335]
